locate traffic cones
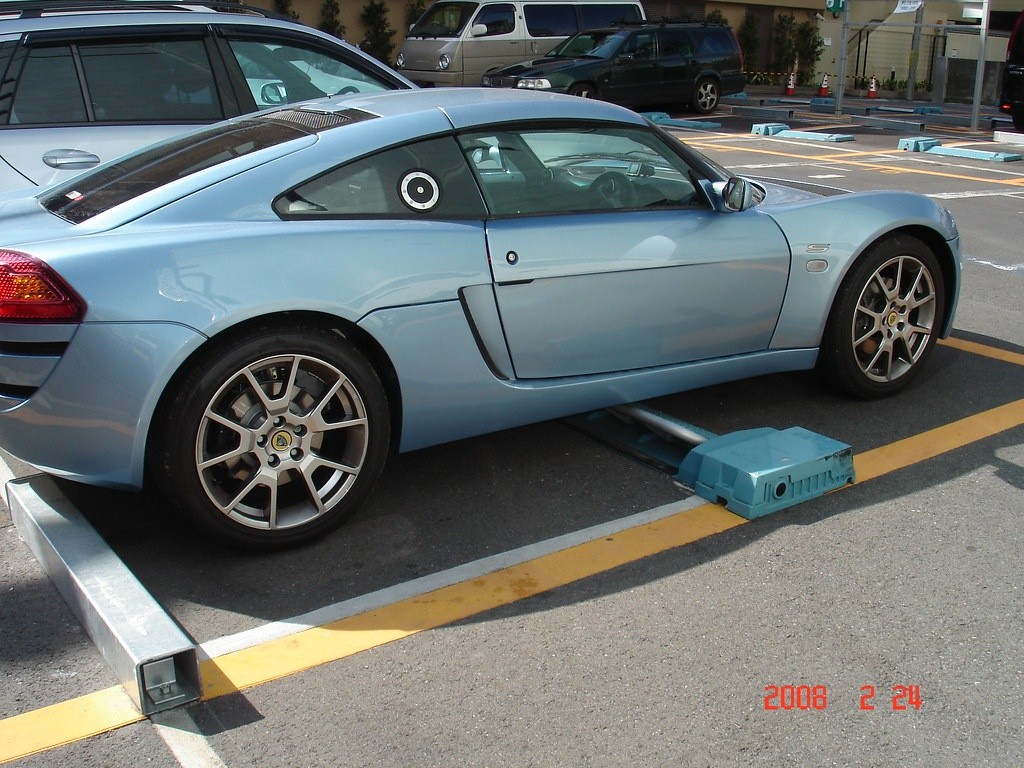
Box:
[816,73,831,97]
[784,72,796,97]
[864,75,881,99]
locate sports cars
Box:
[0,86,963,554]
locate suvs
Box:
[997,10,1024,131]
[479,18,748,114]
[0,0,421,208]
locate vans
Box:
[393,0,648,89]
